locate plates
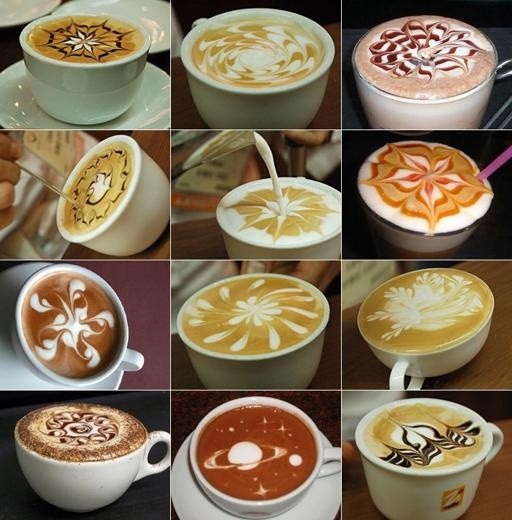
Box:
[1,263,125,390]
[1,130,97,260]
[52,0,170,54]
[170,430,341,519]
[0,0,66,28]
[0,59,170,129]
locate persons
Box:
[1,132,23,236]
[340,389,405,481]
[171,259,340,338]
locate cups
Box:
[179,9,337,128]
[17,15,152,127]
[215,177,341,260]
[350,13,499,130]
[56,135,170,257]
[175,273,329,391]
[12,405,171,510]
[356,266,495,390]
[355,396,505,518]
[186,397,342,518]
[355,144,494,258]
[9,262,145,386]
